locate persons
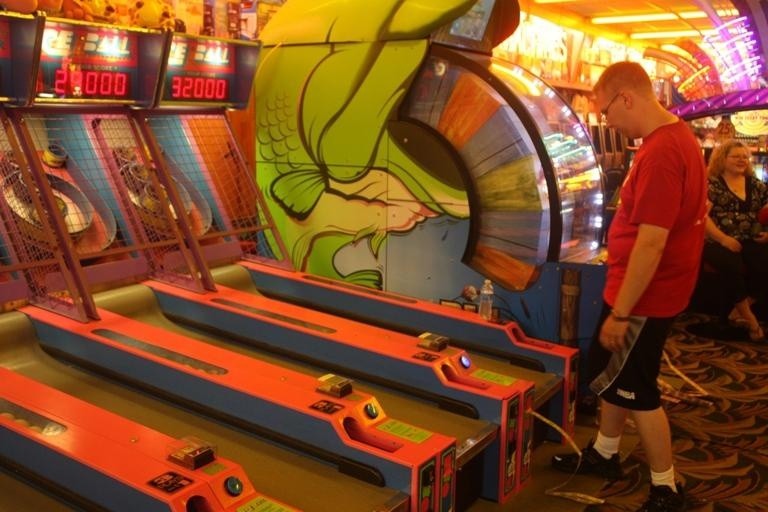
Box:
[704,140,767,343]
[551,61,708,512]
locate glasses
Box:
[601,92,620,117]
[727,155,749,160]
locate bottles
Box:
[478,279,494,322]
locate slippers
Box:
[728,314,765,342]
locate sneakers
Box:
[637,482,685,512]
[552,443,624,477]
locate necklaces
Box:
[726,177,738,193]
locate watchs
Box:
[609,309,630,322]
